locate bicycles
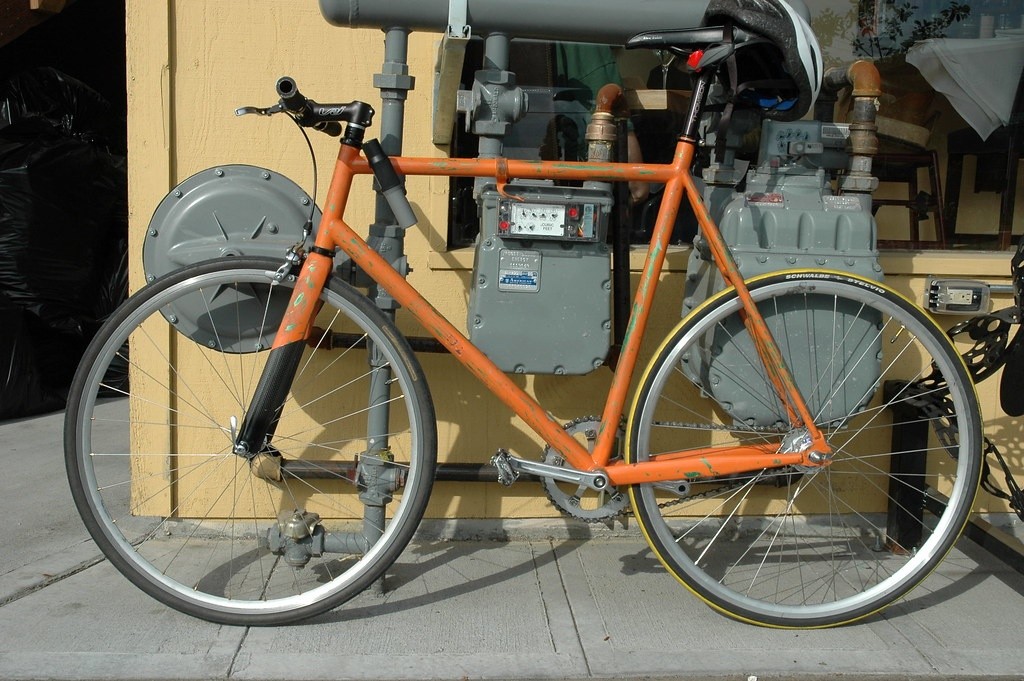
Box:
[58,21,986,631]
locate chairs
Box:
[942,75,1024,245]
[821,93,947,248]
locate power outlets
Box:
[947,290,973,305]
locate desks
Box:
[879,33,1024,247]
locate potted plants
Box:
[851,0,970,125]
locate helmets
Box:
[698,0,824,121]
